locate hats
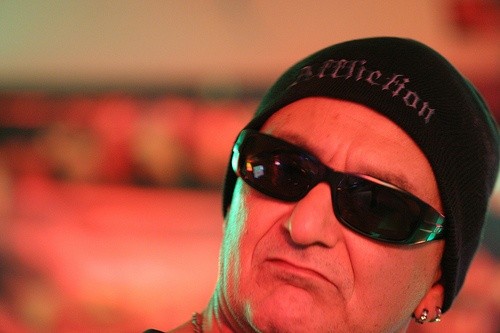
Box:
[223,37,500,313]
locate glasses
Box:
[230,129,447,246]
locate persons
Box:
[145,36,500,333]
